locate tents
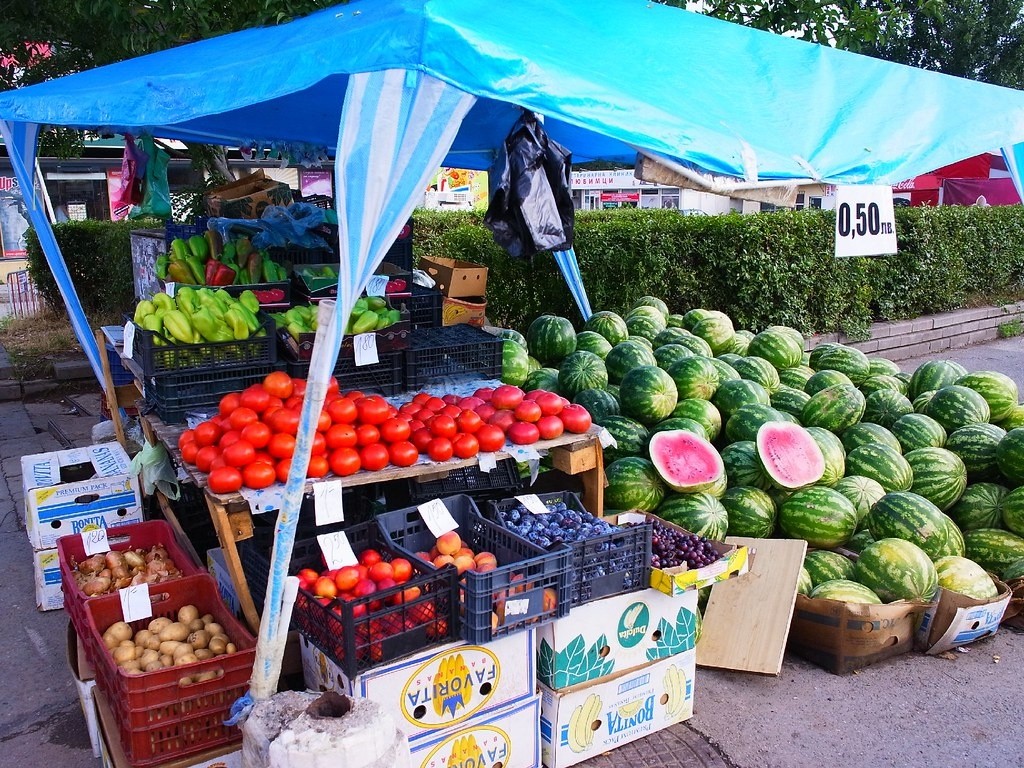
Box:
[1,0,1024,729]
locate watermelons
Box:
[696,297,1024,650]
[500,295,737,543]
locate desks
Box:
[134,397,601,631]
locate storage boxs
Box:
[31,546,68,611]
[605,505,748,596]
[288,524,460,680]
[289,262,411,301]
[122,309,276,375]
[405,322,503,391]
[278,318,411,362]
[175,279,289,306]
[391,284,441,329]
[20,443,145,549]
[492,484,646,601]
[284,349,403,397]
[418,255,488,296]
[91,683,243,768]
[375,495,569,643]
[83,572,258,768]
[142,356,287,426]
[444,296,488,326]
[54,520,199,668]
[300,573,1013,768]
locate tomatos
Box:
[178,371,592,493]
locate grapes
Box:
[623,518,721,572]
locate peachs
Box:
[415,530,557,635]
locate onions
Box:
[69,546,182,597]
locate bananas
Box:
[448,733,485,768]
[432,653,472,715]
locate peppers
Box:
[267,297,400,345]
[133,288,268,368]
[154,229,287,286]
[301,266,338,278]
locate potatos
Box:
[101,604,243,753]
